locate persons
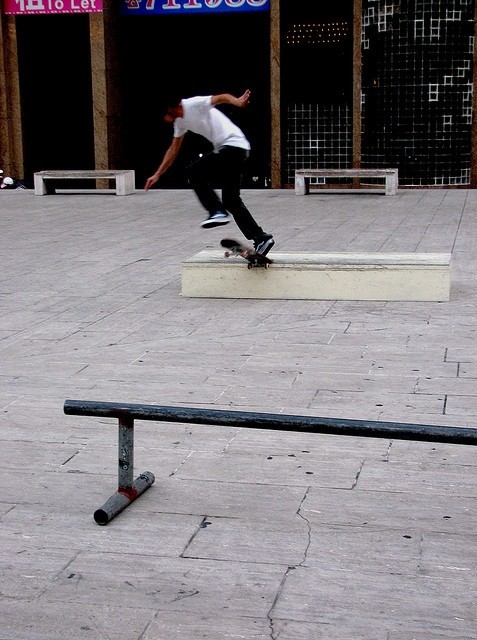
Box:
[143,88,275,257]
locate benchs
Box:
[33,170,136,196]
[294,168,399,196]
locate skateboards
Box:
[220,238,272,269]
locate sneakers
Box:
[200,209,231,229]
[253,234,274,259]
